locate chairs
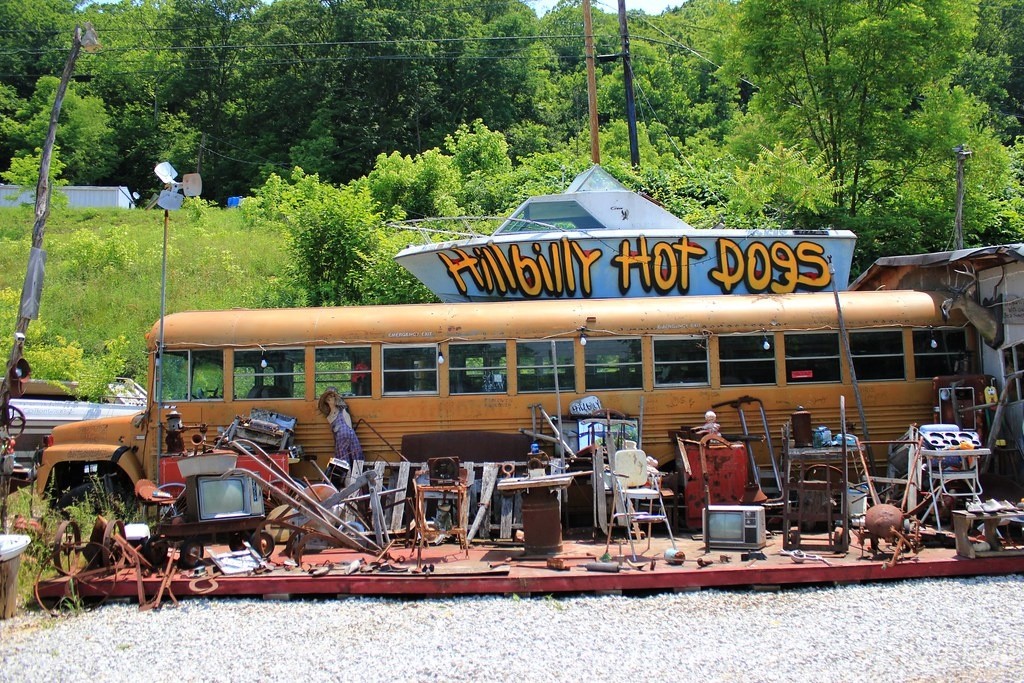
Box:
[248,384,266,397]
[413,470,470,558]
[261,386,281,397]
[607,471,677,564]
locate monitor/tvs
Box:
[700,505,767,551]
[183,470,265,524]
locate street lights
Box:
[10,23,100,383]
[598,53,643,171]
[954,143,973,251]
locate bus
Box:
[28,296,953,520]
